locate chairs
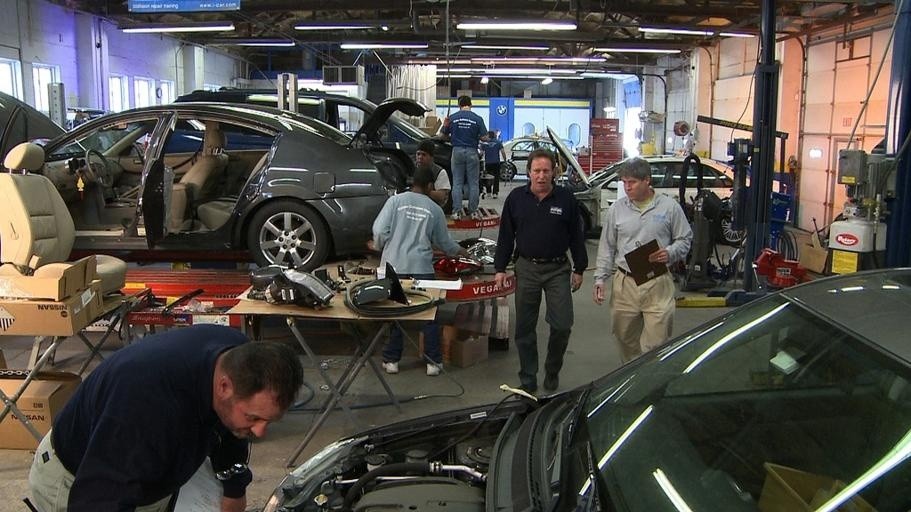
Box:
[179,129,229,209]
[1,143,127,296]
[197,153,269,226]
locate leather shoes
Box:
[543,371,559,390]
[514,382,538,399]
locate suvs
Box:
[171,88,484,216]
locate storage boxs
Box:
[0,281,105,336]
[0,371,83,450]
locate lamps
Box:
[340,39,428,49]
[456,21,577,31]
[117,21,235,33]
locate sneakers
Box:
[425,362,444,376]
[381,360,400,374]
[492,193,498,199]
[450,211,462,221]
[469,211,482,221]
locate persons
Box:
[479,131,506,199]
[495,129,504,146]
[371,167,469,376]
[439,95,488,221]
[29,324,303,512]
[414,140,451,208]
[495,149,588,399]
[592,159,693,365]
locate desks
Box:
[0,294,131,443]
[225,286,445,469]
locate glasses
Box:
[215,439,253,481]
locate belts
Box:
[617,266,633,278]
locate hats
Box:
[415,139,436,156]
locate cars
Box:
[545,125,788,245]
[25,97,432,273]
[499,136,561,182]
[263,268,911,512]
